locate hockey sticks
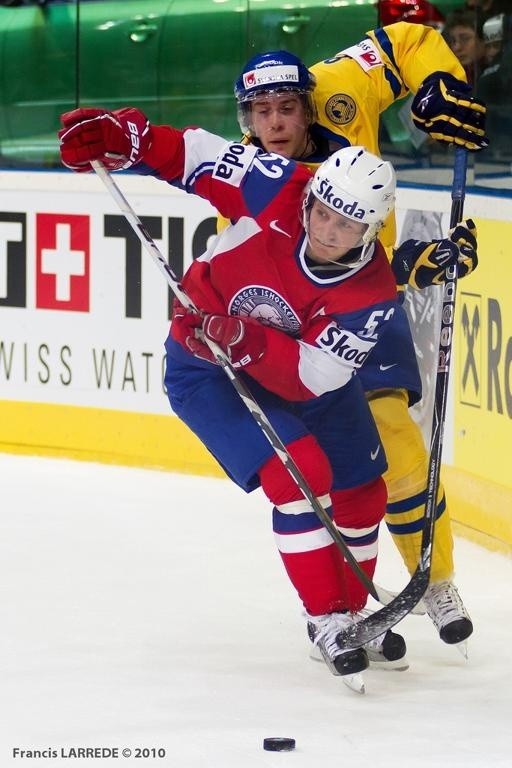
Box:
[93,162,432,616]
[334,147,468,649]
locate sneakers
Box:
[423,582,472,643]
[308,610,406,676]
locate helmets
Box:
[237,51,316,141]
[296,147,396,250]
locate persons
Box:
[444,0,512,164]
[56,106,400,676]
[213,21,487,644]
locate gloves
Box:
[392,220,478,290]
[411,72,489,151]
[55,106,154,174]
[174,296,267,368]
